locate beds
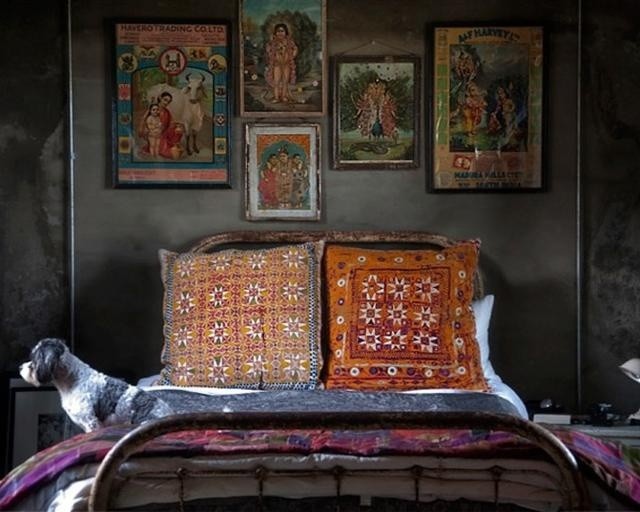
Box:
[49,227,590,510]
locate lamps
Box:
[618,358,640,423]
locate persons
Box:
[136,91,184,159]
[256,152,278,208]
[291,151,308,209]
[144,104,162,156]
[267,145,296,209]
[493,87,518,143]
[264,22,302,104]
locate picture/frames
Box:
[239,119,324,224]
[327,50,425,175]
[8,385,82,474]
[107,14,234,192]
[422,16,550,198]
[230,1,332,123]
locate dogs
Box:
[18,336,234,434]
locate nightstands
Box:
[570,421,640,471]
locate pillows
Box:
[155,237,329,396]
[323,235,493,396]
